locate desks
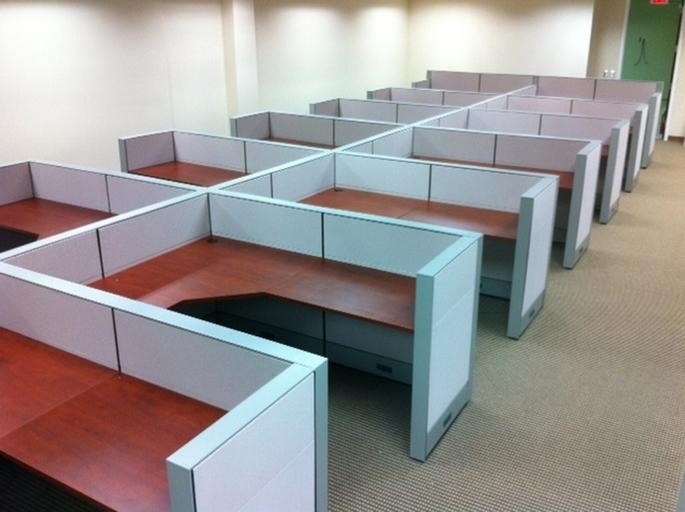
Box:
[0,67,667,512]
